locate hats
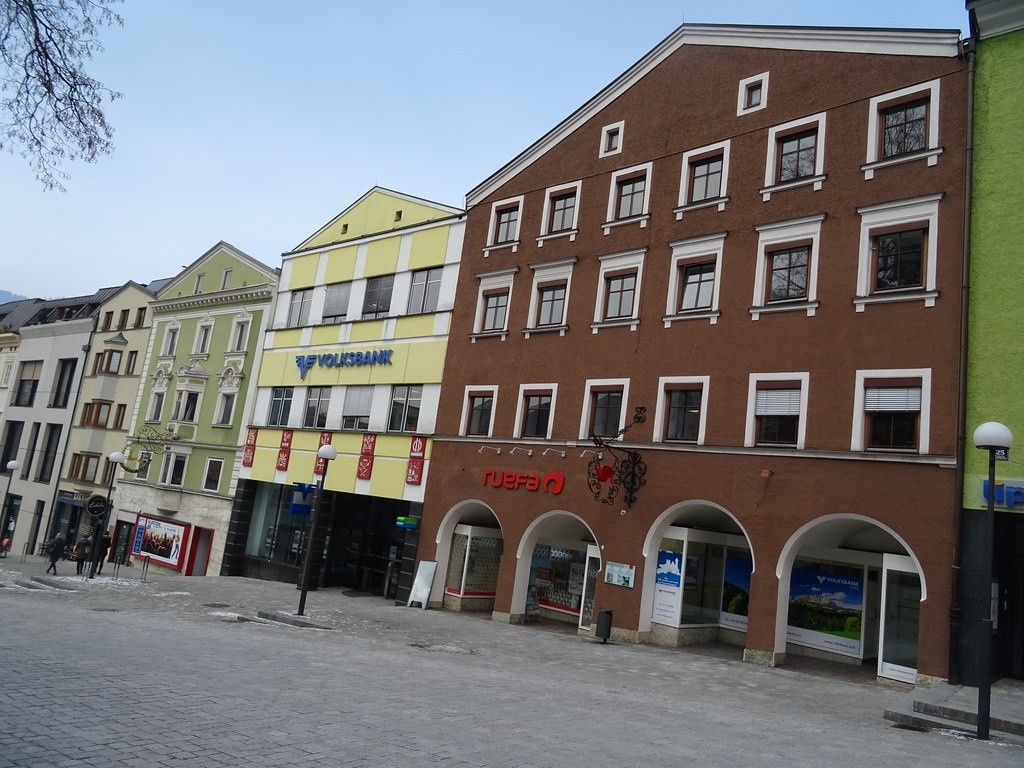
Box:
[104,531,109,535]
[83,534,90,538]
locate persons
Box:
[75,533,91,576]
[46,533,64,575]
[90,531,112,574]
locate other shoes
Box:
[47,570,50,573]
[52,572,57,574]
[89,576,93,579]
[97,572,100,575]
[75,572,82,575]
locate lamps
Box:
[478,446,500,454]
[580,449,602,460]
[510,447,532,456]
[543,448,565,458]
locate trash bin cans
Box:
[595,607,615,645]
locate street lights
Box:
[297,446,340,618]
[0,459,19,526]
[88,452,126,581]
[970,423,1012,740]
